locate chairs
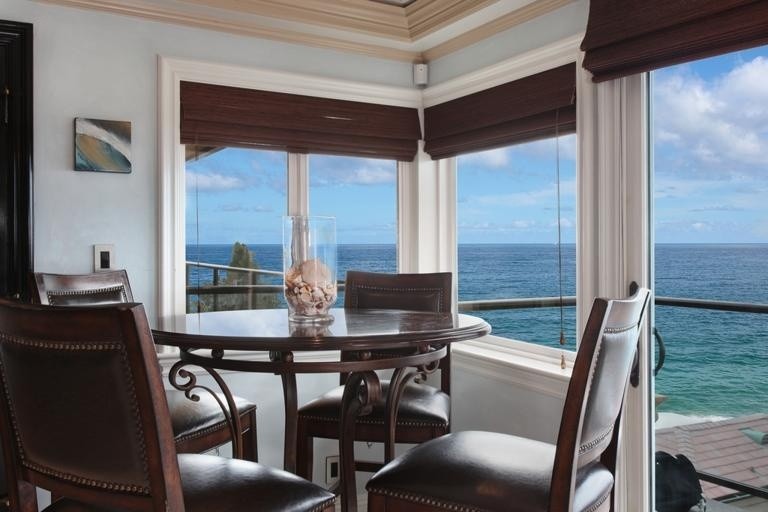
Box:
[29,271,264,474]
[282,271,460,478]
[2,296,344,512]
[360,276,652,512]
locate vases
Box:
[285,216,339,327]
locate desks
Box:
[135,302,497,512]
